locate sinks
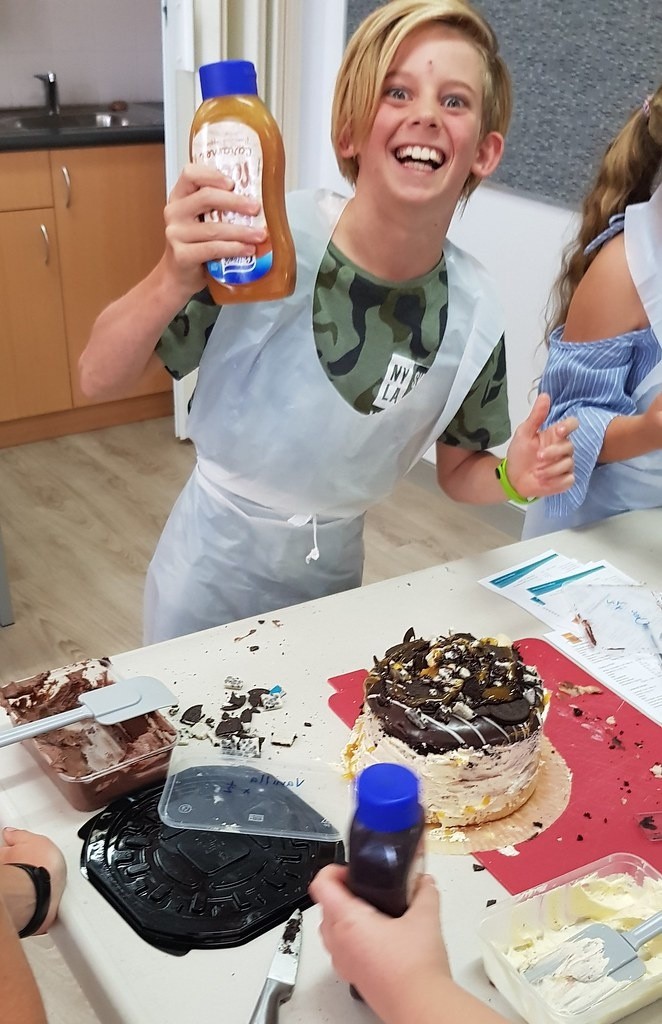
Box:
[0,100,169,137]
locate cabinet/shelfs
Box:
[0,101,173,448]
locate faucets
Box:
[32,70,62,114]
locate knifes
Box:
[246,909,303,1024]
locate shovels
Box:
[523,910,662,1015]
[0,675,180,747]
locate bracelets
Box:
[3,862,51,939]
[495,458,541,506]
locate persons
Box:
[75,0,580,647]
[0,826,68,1024]
[307,864,515,1024]
[522,87,662,538]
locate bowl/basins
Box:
[474,852,662,1023]
[2,659,179,814]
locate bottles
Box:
[346,762,430,1003]
[189,61,298,304]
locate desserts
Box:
[1,666,171,808]
[489,875,662,1023]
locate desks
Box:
[0,506,662,1024]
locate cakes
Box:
[342,631,553,826]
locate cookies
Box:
[166,676,298,760]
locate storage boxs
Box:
[3,658,182,815]
[475,851,662,1024]
